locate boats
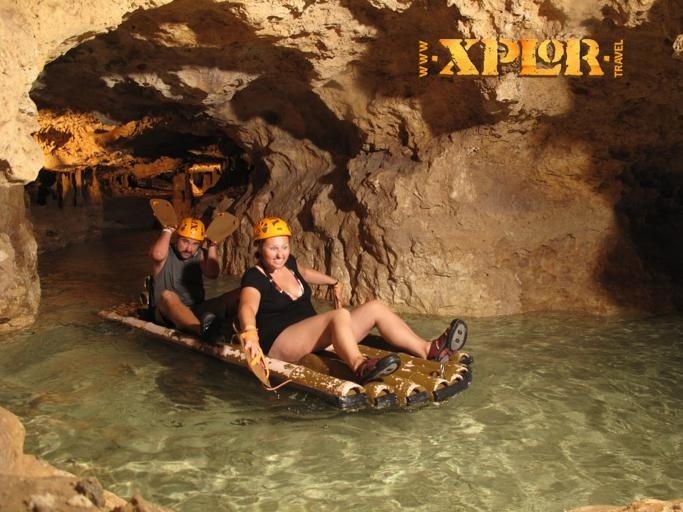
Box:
[99,298,473,415]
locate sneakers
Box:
[347,353,401,384]
[427,319,467,362]
[199,311,221,341]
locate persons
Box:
[150,218,241,334]
[236,217,466,383]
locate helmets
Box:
[253,216,291,243]
[176,218,205,240]
